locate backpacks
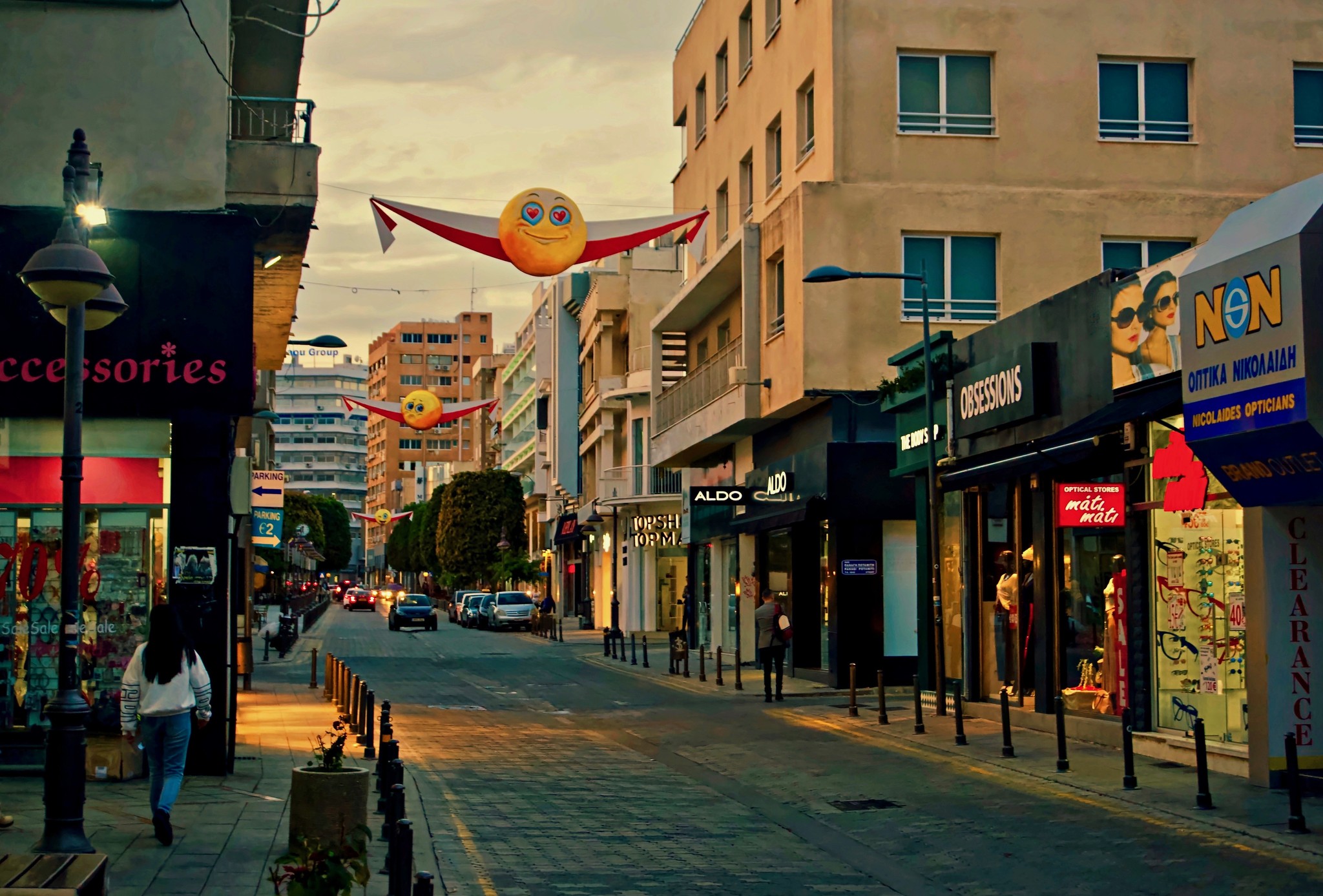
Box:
[770,604,793,650]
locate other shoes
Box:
[775,693,783,700]
[765,697,772,702]
[152,809,173,846]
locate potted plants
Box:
[288,715,370,859]
[877,353,968,414]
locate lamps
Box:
[510,471,535,484]
[729,366,772,389]
[288,335,348,347]
[254,249,282,269]
[254,410,280,420]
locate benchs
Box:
[0,853,109,896]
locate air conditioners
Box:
[434,428,441,434]
[275,362,384,503]
[415,429,424,434]
[434,364,450,372]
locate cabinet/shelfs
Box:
[656,578,684,631]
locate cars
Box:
[371,582,438,632]
[447,589,538,632]
[287,577,378,611]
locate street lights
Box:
[15,130,131,852]
[496,520,512,590]
[579,487,624,639]
[801,257,946,716]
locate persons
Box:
[531,585,542,602]
[1102,554,1124,712]
[294,587,302,596]
[1108,270,1181,390]
[485,584,492,591]
[497,584,501,591]
[433,582,441,595]
[120,603,214,846]
[992,550,1018,696]
[423,582,429,594]
[1020,544,1038,697]
[539,592,557,614]
[318,579,330,600]
[755,588,792,702]
[678,575,689,633]
